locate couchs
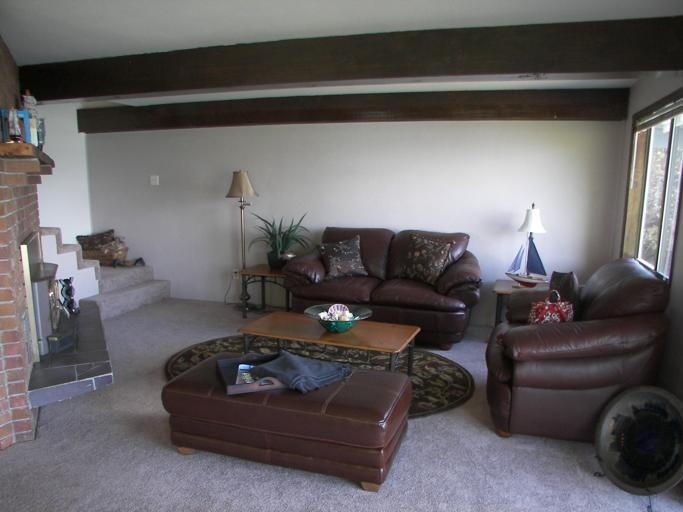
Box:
[486,258,670,450]
[280,225,484,352]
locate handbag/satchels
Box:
[528,289,574,326]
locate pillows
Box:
[315,233,370,282]
[397,230,455,283]
[546,267,582,320]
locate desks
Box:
[236,264,296,312]
[493,279,561,324]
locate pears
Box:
[339,316,349,321]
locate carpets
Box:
[163,325,474,422]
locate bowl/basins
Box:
[303,304,372,333]
[594,384,682,495]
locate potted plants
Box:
[247,208,316,268]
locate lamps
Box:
[515,203,545,286]
[225,168,260,317]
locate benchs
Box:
[160,352,420,492]
